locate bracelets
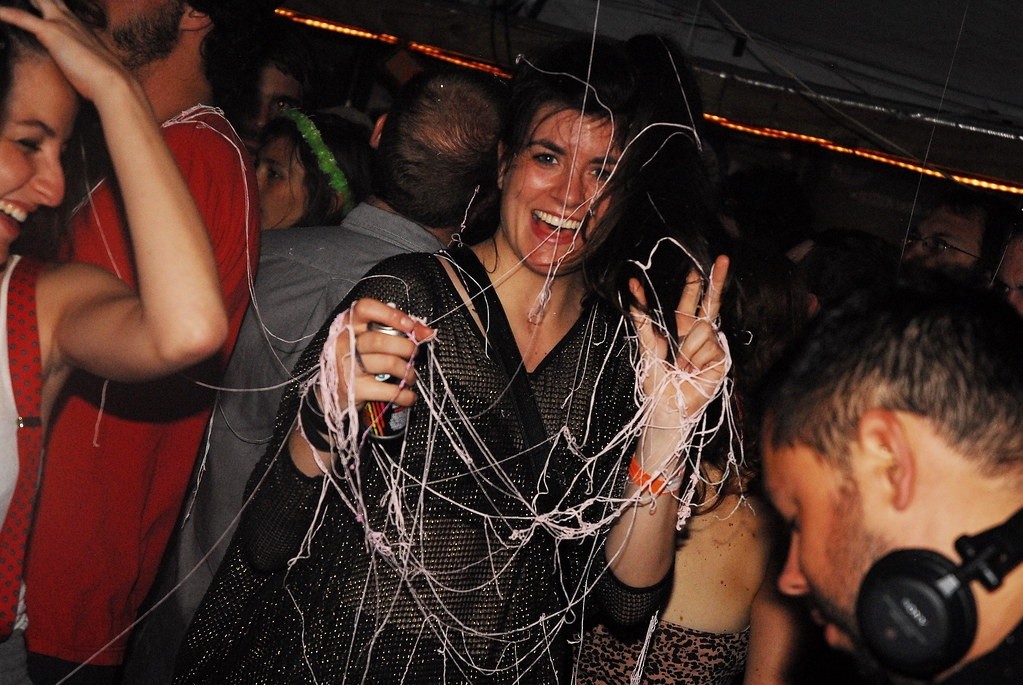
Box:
[629,452,687,496]
[296,381,349,456]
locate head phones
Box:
[853,506,1023,679]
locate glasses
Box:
[899,231,983,261]
[991,279,1023,302]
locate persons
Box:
[0,0,228,685]
[23,9,261,685]
[760,277,1023,685]
[255,108,383,230]
[570,204,804,685]
[169,38,733,685]
[339,79,384,135]
[626,34,704,130]
[231,33,319,165]
[122,65,512,684]
[998,225,1023,316]
[901,191,1016,290]
[785,227,939,336]
